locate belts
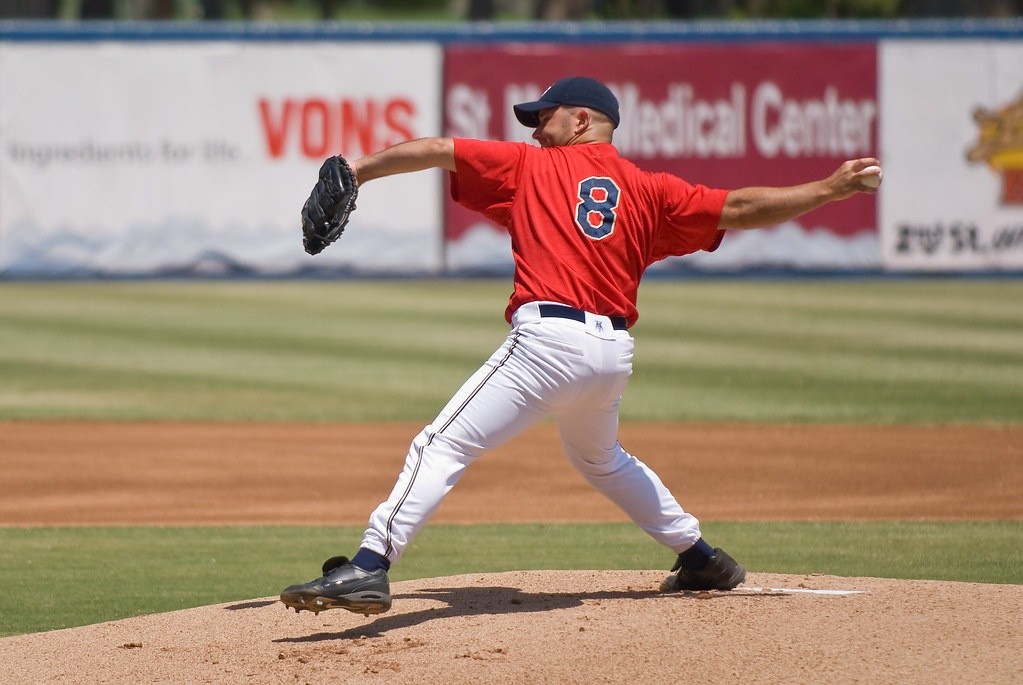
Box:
[541,303,631,330]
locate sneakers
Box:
[660,546,747,594]
[281,557,392,617]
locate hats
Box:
[512,78,620,129]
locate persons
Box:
[279,76,881,618]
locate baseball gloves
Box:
[298,152,359,257]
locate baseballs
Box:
[861,165,884,189]
